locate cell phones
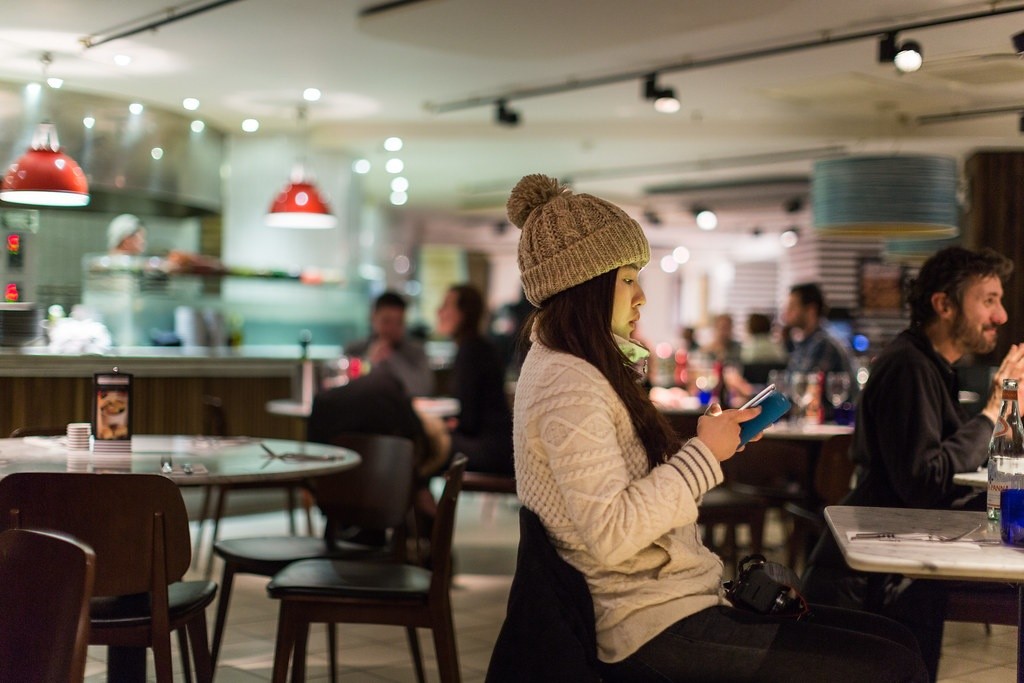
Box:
[739,384,776,410]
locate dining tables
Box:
[754,420,857,504]
[823,504,1024,682]
[0,435,362,683]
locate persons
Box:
[89,212,174,274]
[506,174,929,683]
[344,284,536,464]
[682,246,1024,513]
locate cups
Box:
[687,360,716,395]
[1000,489,1024,547]
[826,371,854,425]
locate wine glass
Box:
[767,369,822,424]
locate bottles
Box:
[987,379,1024,520]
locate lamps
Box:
[496,4,1024,127]
[0,50,91,208]
[264,99,335,228]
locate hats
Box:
[507,174,649,308]
[108,213,139,247]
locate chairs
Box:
[0,363,512,683]
